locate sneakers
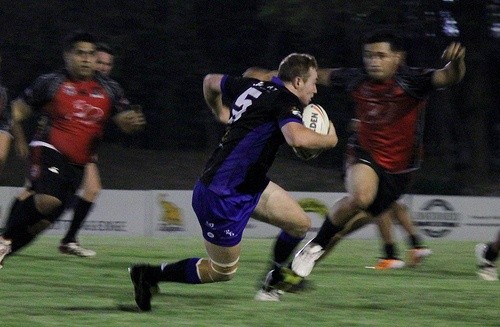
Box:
[263,260,305,293]
[375,258,405,270]
[407,248,431,259]
[0,235,12,263]
[60,242,96,256]
[475,242,499,281]
[291,237,326,277]
[255,288,279,301]
[130,264,158,310]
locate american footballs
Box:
[292,104,330,162]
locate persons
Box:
[473,230,500,282]
[0,33,148,268]
[243,28,466,292]
[129,52,337,312]
[345,116,433,269]
